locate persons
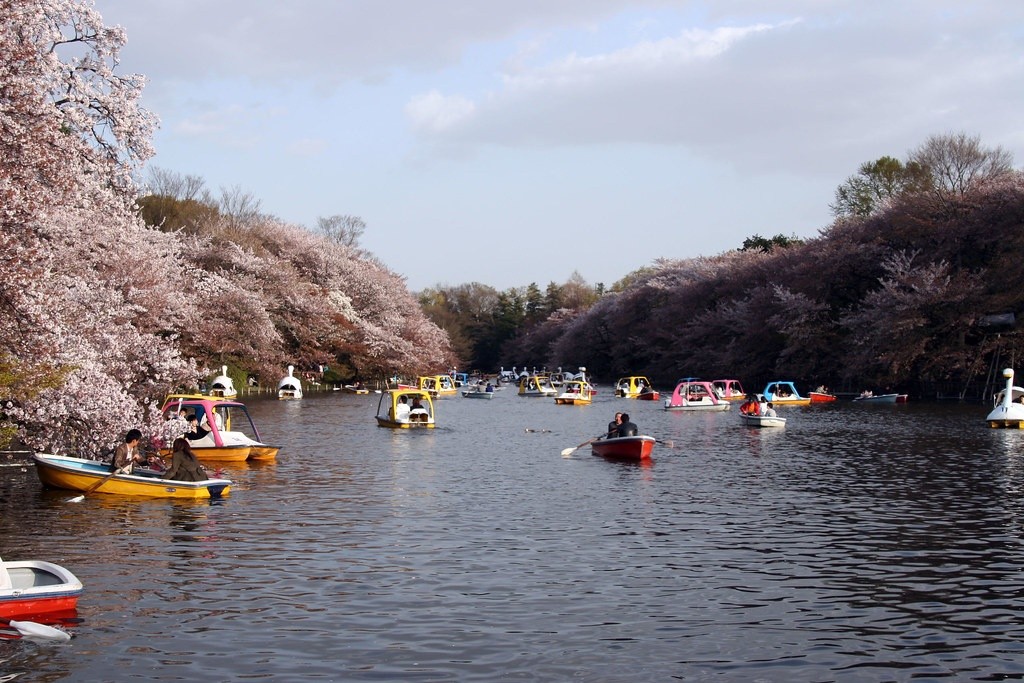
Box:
[396,395,410,423]
[108,429,155,476]
[159,438,210,481]
[175,407,223,440]
[429,372,874,440]
[410,397,424,412]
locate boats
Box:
[278,365,302,401]
[985,369,1024,429]
[462,390,493,398]
[209,366,237,401]
[469,366,597,395]
[591,434,655,460]
[554,380,591,406]
[346,384,369,395]
[388,366,487,395]
[664,376,747,412]
[854,394,909,404]
[0,554,83,639]
[754,382,811,407]
[375,387,436,428]
[807,391,836,403]
[160,395,282,461]
[615,376,660,400]
[32,451,232,500]
[740,412,786,428]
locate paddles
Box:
[561,429,617,455]
[656,439,674,449]
[68,457,137,502]
